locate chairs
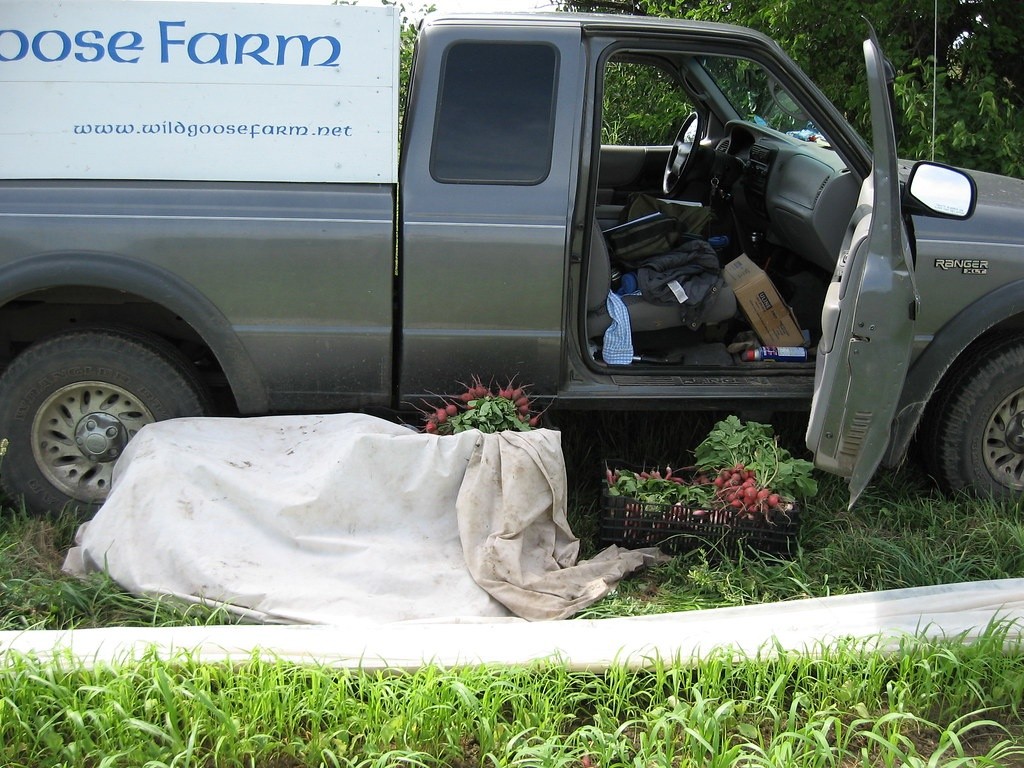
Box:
[586,217,737,339]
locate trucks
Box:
[0,2,1023,518]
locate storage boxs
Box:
[723,251,807,348]
[596,459,802,562]
